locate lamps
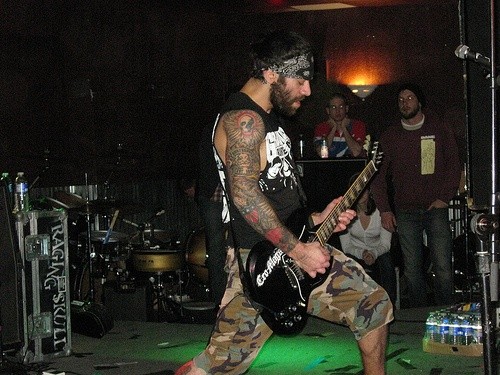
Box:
[348,84,378,101]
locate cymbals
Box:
[59,190,84,207]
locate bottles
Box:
[319,134,329,159]
[295,134,306,160]
[0,172,15,211]
[15,172,30,213]
[426,301,484,345]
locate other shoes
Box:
[400,294,437,309]
[438,295,452,308]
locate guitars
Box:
[245,134,383,335]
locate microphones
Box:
[454,44,491,67]
[469,213,500,235]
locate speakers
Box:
[101,280,155,321]
[0,181,24,352]
[296,157,369,235]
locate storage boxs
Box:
[422,338,485,357]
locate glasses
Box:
[329,104,345,110]
[397,95,415,103]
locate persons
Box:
[369,84,461,309]
[313,93,367,160]
[149,29,394,375]
[339,172,396,309]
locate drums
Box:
[130,230,175,249]
[75,254,173,322]
[133,249,184,273]
[184,225,209,294]
[75,231,131,261]
